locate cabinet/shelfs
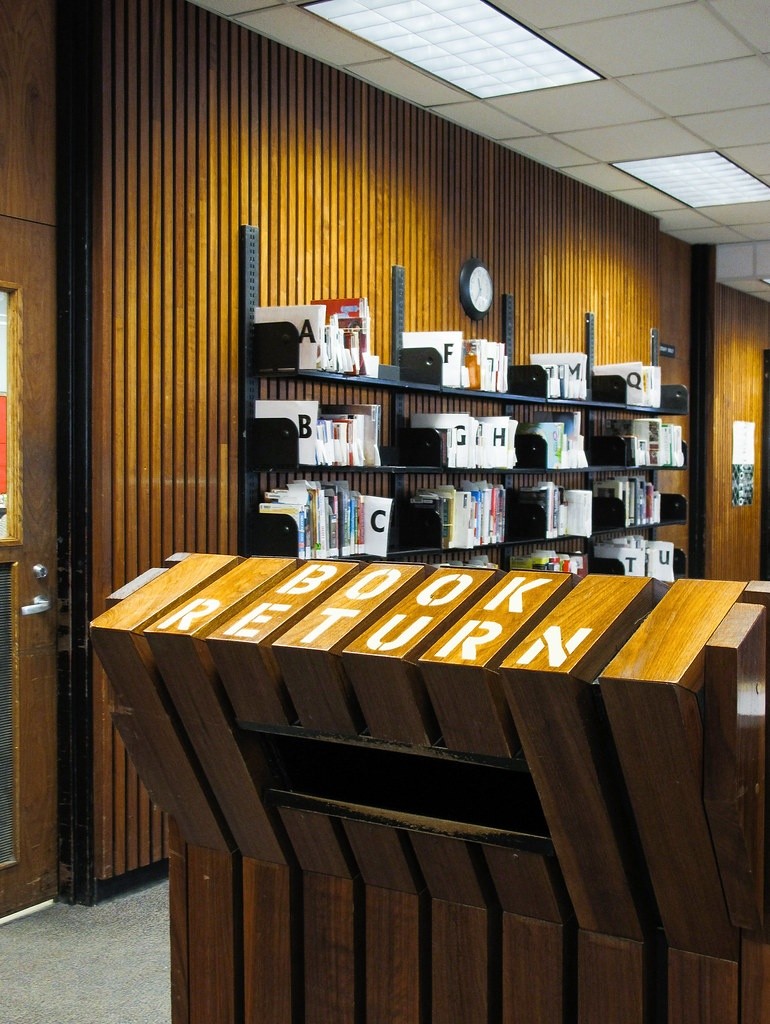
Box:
[238,225,691,580]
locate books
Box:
[254,298,371,375]
[448,555,499,569]
[594,362,662,409]
[256,401,382,467]
[604,419,684,466]
[520,482,592,536]
[593,476,661,527]
[399,480,505,549]
[530,353,587,401]
[412,413,518,469]
[516,410,588,469]
[509,549,589,578]
[594,536,675,583]
[258,480,394,559]
[402,331,508,393]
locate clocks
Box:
[459,258,495,321]
